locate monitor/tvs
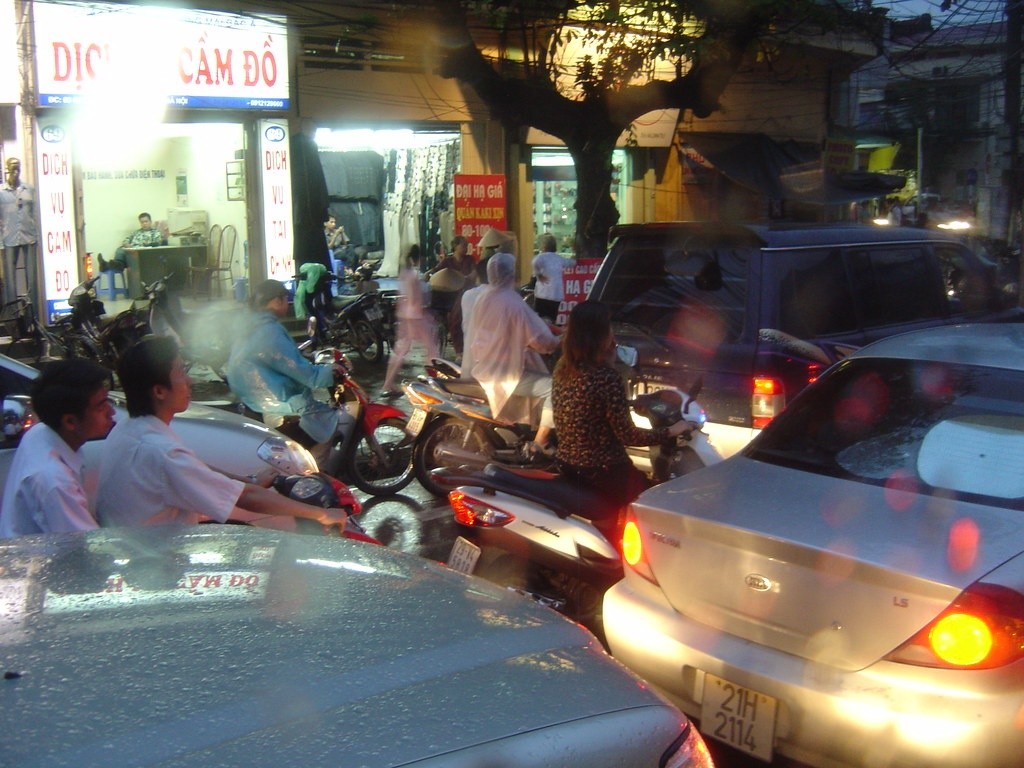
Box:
[168,208,207,236]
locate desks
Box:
[121,244,208,300]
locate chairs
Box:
[328,247,346,297]
[185,223,237,301]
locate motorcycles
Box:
[338,258,412,355]
[400,322,565,500]
[128,269,242,384]
[426,342,727,627]
[296,347,420,497]
[69,275,150,377]
[290,269,387,365]
[246,470,382,546]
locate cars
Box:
[599,321,1023,767]
[0,526,715,768]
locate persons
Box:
[529,233,576,322]
[226,279,350,474]
[0,335,348,541]
[552,299,702,510]
[378,227,518,401]
[461,251,566,461]
[98,213,164,274]
[324,212,368,275]
[0,158,42,329]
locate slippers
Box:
[381,391,403,397]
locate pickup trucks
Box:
[902,194,941,225]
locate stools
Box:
[95,271,129,302]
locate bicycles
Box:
[0,287,115,393]
[423,272,471,363]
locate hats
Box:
[476,228,513,247]
[428,267,466,292]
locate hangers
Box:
[317,144,374,152]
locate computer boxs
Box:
[168,235,208,246]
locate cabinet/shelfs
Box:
[226,159,246,202]
[552,193,576,227]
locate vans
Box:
[0,353,321,537]
[581,218,1012,472]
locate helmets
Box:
[255,279,296,302]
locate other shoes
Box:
[97,253,107,272]
[524,440,556,461]
[109,260,124,272]
